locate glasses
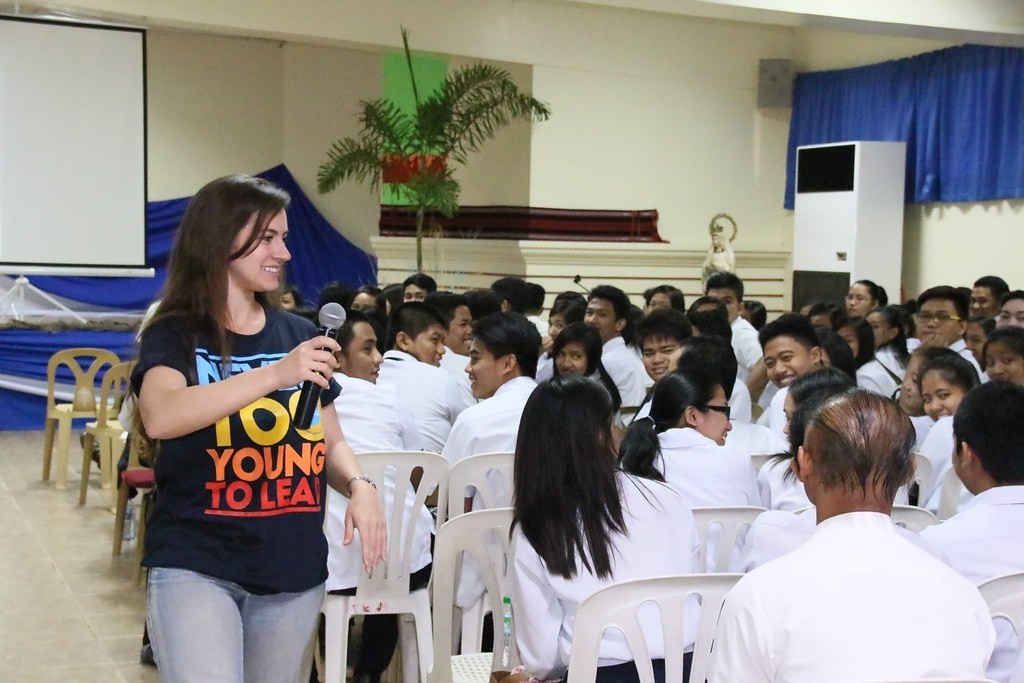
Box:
[916,310,962,326]
[696,404,730,420]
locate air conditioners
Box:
[791,139,906,315]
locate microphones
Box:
[292,303,347,430]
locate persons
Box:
[441,309,542,656]
[982,325,1024,386]
[701,232,736,294]
[912,379,1024,683]
[747,312,826,432]
[625,336,785,452]
[507,372,702,683]
[803,275,1024,506]
[707,389,998,683]
[907,354,975,516]
[129,171,391,683]
[756,365,859,513]
[308,306,436,683]
[545,323,622,427]
[616,369,762,574]
[274,273,766,422]
[743,387,970,576]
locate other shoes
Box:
[81,432,101,459]
[354,671,380,683]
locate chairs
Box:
[44,347,1024,683]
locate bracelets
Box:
[348,477,377,493]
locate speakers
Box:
[757,59,792,108]
